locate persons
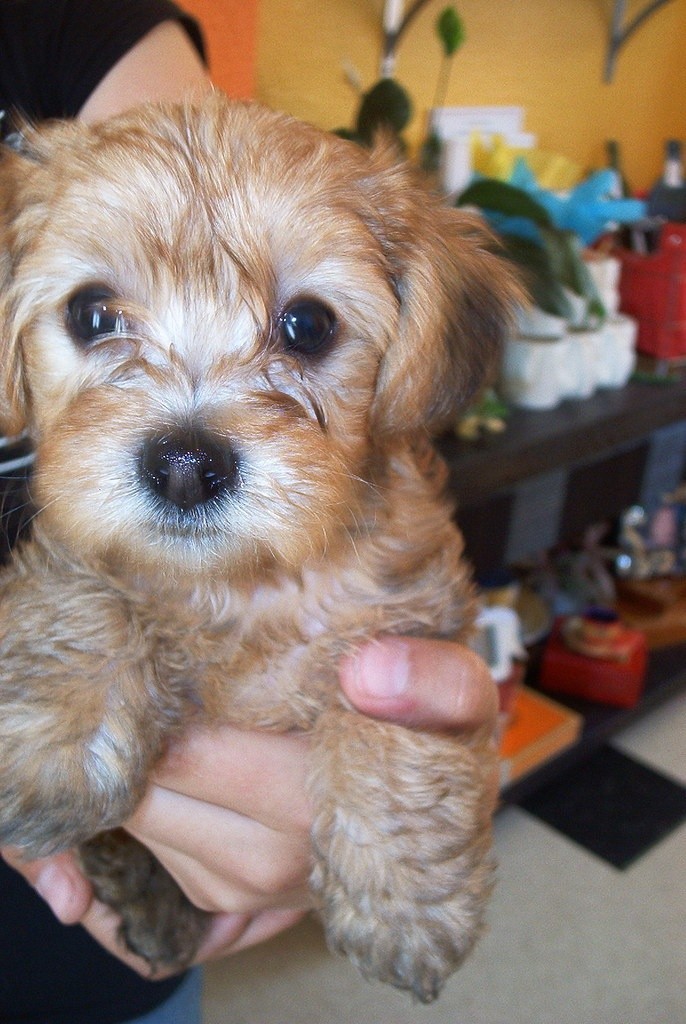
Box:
[0,0,502,1024]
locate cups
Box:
[501,258,636,408]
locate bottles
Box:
[646,141,686,250]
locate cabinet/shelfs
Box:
[431,373,685,590]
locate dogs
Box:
[1,80,534,1005]
